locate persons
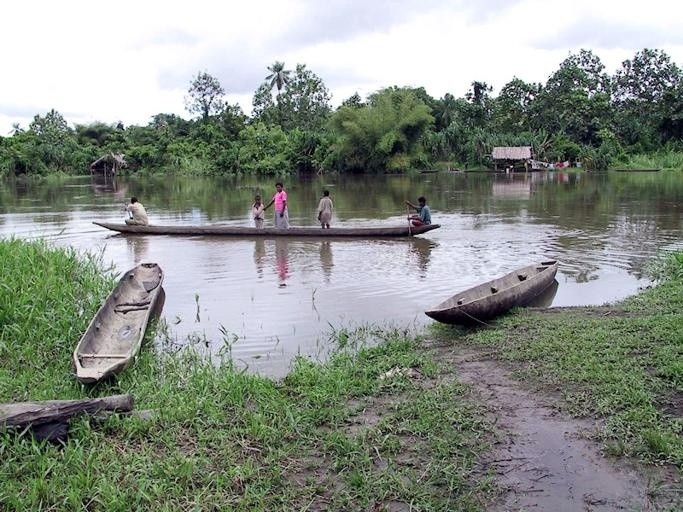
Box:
[404,196,431,227]
[262,183,288,228]
[317,191,333,228]
[252,195,264,229]
[123,196,147,225]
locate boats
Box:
[71,262,164,382]
[424,258,560,328]
[615,168,662,172]
[91,219,442,237]
